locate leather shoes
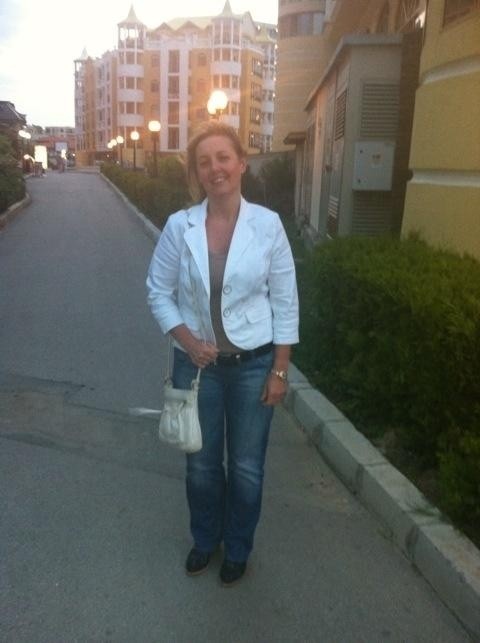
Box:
[186,551,209,576]
[219,559,247,587]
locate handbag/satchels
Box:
[158,377,203,454]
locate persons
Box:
[146,119,301,583]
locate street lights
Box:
[130,131,140,173]
[107,136,124,168]
[148,121,161,177]
[19,130,32,173]
[207,91,229,121]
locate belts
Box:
[209,342,274,369]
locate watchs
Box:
[271,369,288,380]
